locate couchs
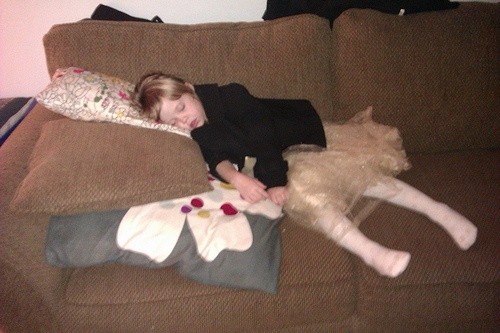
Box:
[0,3,500,332]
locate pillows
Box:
[43,169,284,296]
[33,66,192,139]
[11,118,216,218]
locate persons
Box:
[130,71,479,279]
